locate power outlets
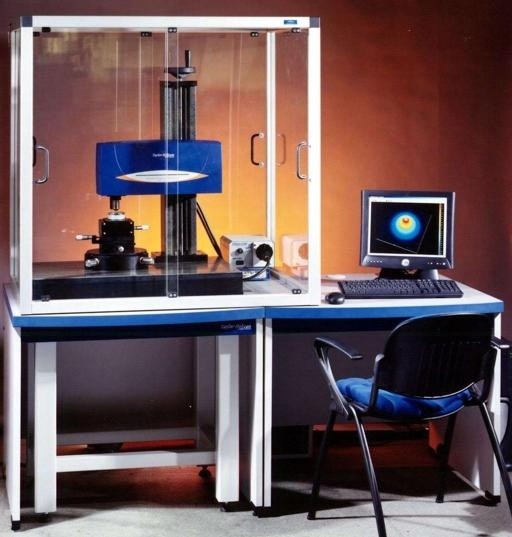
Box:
[252,241,275,268]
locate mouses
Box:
[325,292,345,304]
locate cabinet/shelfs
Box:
[5,14,324,315]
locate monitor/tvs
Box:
[360,190,456,280]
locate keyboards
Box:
[338,279,464,299]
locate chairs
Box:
[307,311,512,535]
